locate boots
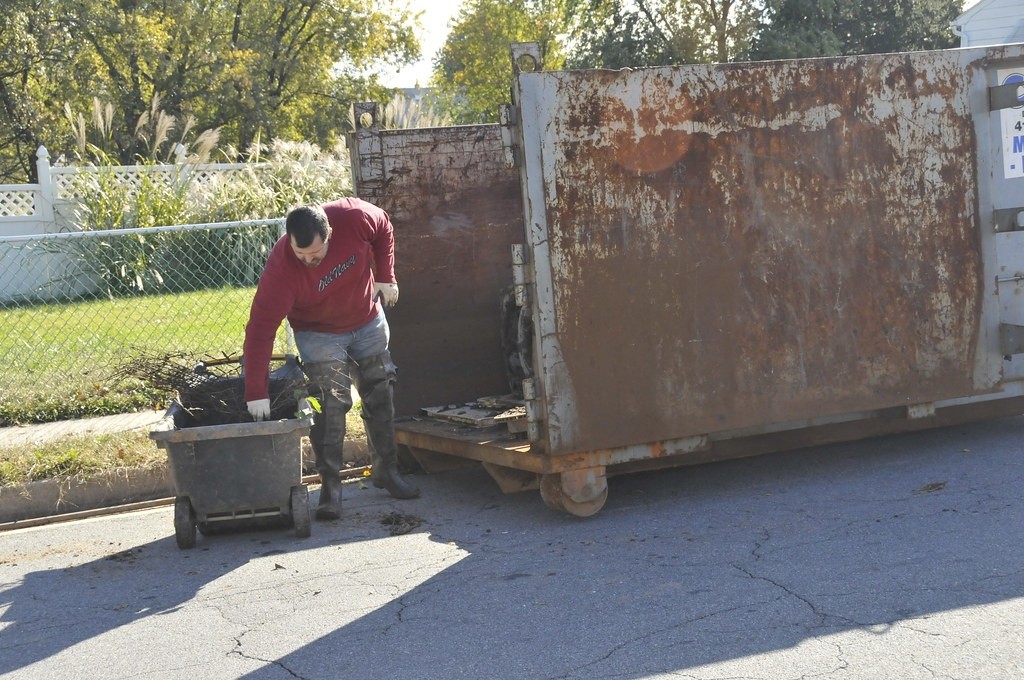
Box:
[350,349,421,498]
[309,358,352,518]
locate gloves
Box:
[246,398,271,422]
[372,282,399,308]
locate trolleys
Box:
[148,355,314,550]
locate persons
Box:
[243,196,421,521]
